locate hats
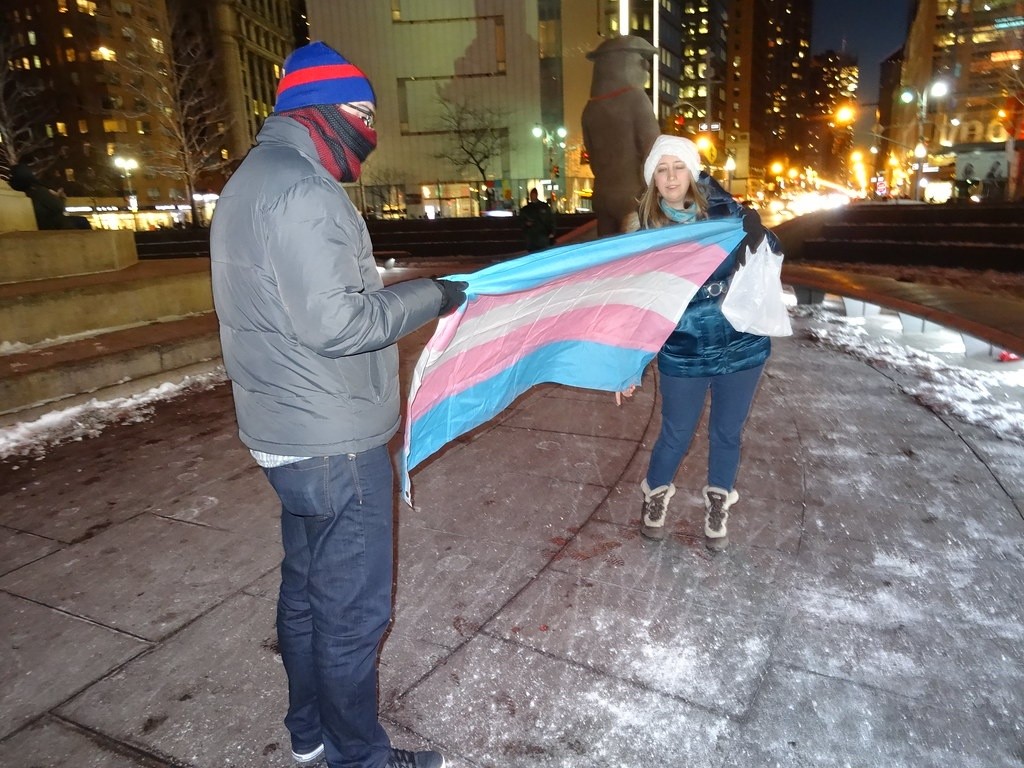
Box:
[274,40,376,114]
[643,134,702,187]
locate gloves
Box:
[734,209,765,269]
[432,275,468,316]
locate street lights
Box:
[724,156,736,193]
[900,78,947,201]
[531,122,567,213]
[115,157,140,232]
[839,106,855,186]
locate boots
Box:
[701,485,739,553]
[639,478,675,541]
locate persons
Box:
[519,188,555,254]
[548,192,561,213]
[210,40,470,768]
[615,135,782,552]
[502,190,516,215]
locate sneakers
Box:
[384,748,445,768]
[291,744,324,762]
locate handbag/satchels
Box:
[721,235,794,338]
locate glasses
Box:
[343,103,375,128]
